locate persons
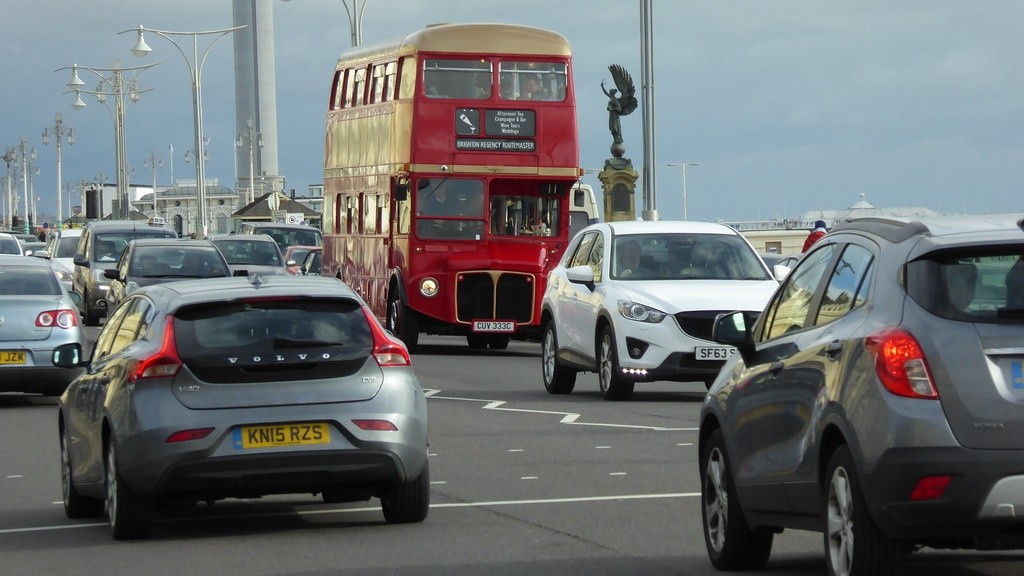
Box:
[617,241,659,277]
[601,82,623,143]
[801,220,828,253]
[419,182,453,226]
[500,75,547,100]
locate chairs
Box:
[272,234,284,247]
[97,241,119,257]
[997,256,1024,312]
[679,244,713,276]
[946,262,978,311]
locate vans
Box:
[72,219,180,326]
[239,222,323,262]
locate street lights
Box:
[9,144,36,234]
[235,118,264,202]
[61,87,159,218]
[42,124,75,230]
[53,62,161,219]
[19,165,40,227]
[63,136,210,224]
[118,24,251,236]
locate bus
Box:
[321,23,585,352]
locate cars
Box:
[34,230,128,293]
[281,245,323,274]
[201,233,289,277]
[0,254,87,397]
[104,239,249,323]
[0,230,50,256]
[697,206,1024,576]
[541,217,781,401]
[57,275,431,539]
